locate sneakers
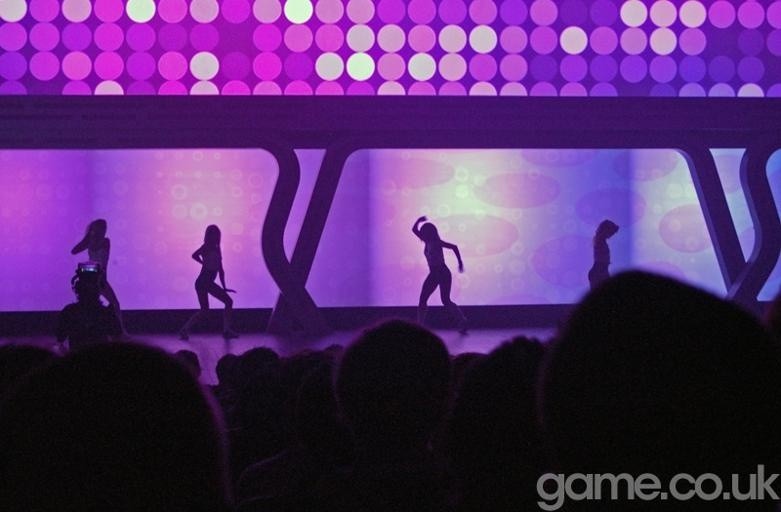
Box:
[177,329,188,340]
[222,327,240,337]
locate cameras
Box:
[77,262,101,277]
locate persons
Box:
[178,224,240,343]
[411,215,473,338]
[70,218,132,340]
[0,268,781,511]
[587,217,621,300]
